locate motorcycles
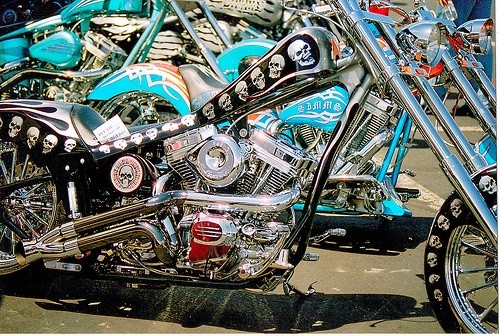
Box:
[1,1,497,334]
